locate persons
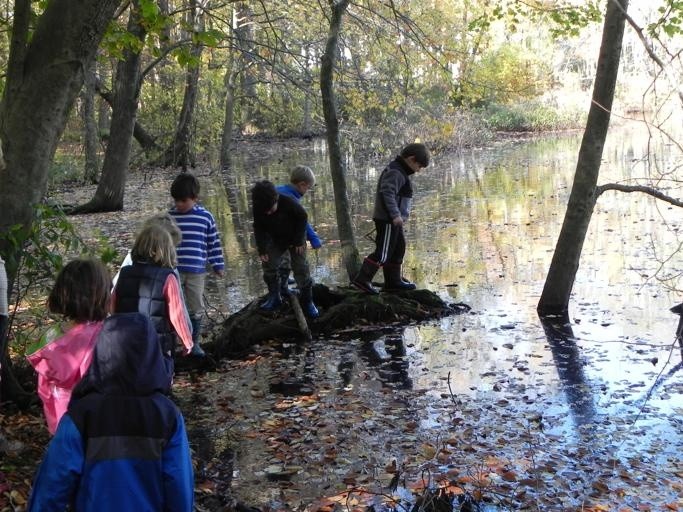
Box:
[110,227,194,359]
[165,173,225,357]
[273,165,321,295]
[28,312,194,511]
[354,143,430,294]
[250,180,320,318]
[27,256,113,434]
[110,213,182,294]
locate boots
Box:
[352,257,378,294]
[280,273,299,296]
[300,288,318,317]
[383,262,416,292]
[259,279,282,310]
[288,277,296,284]
[187,319,204,357]
